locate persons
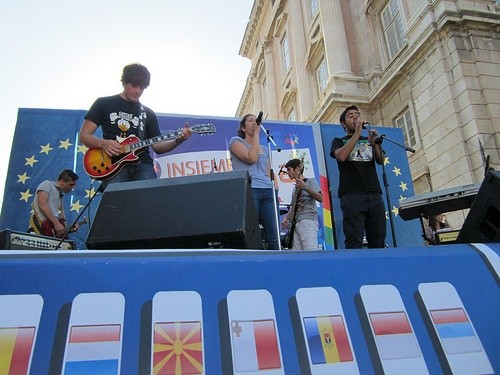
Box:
[79,64,192,191]
[229,113,285,251]
[330,105,387,248]
[424,215,453,246]
[280,159,324,250]
[27,169,88,250]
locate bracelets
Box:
[305,185,310,191]
[175,137,180,145]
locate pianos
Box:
[398,182,481,247]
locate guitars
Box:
[41,216,89,240]
[83,122,216,182]
[283,156,309,249]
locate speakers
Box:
[456,170,500,243]
[84,170,263,250]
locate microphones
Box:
[213,158,217,171]
[362,121,369,124]
[256,111,263,125]
[278,163,285,175]
